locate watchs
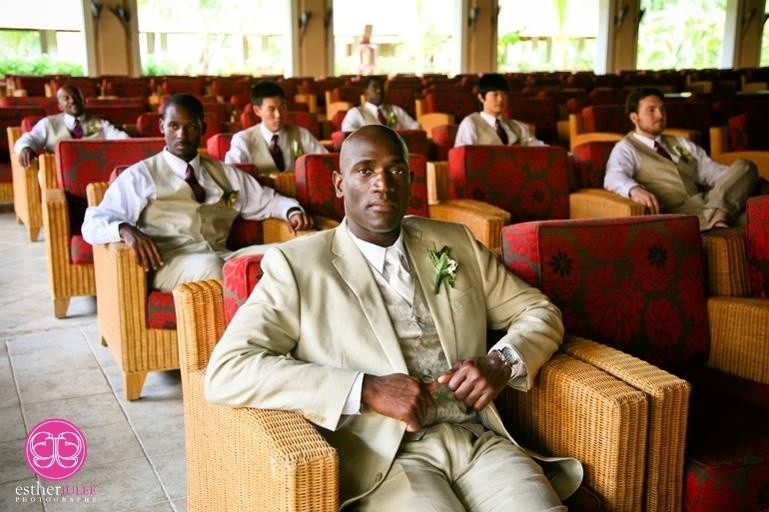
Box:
[491,346,520,367]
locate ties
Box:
[377,108,386,125]
[496,119,508,145]
[654,141,670,161]
[384,247,414,306]
[70,119,83,139]
[269,135,285,172]
[184,164,205,202]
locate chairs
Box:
[2,65,768,511]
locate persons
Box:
[203,123,587,512]
[78,93,315,295]
[603,86,758,229]
[341,75,423,130]
[15,83,132,169]
[454,72,550,147]
[223,80,331,182]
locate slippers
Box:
[701,221,729,233]
